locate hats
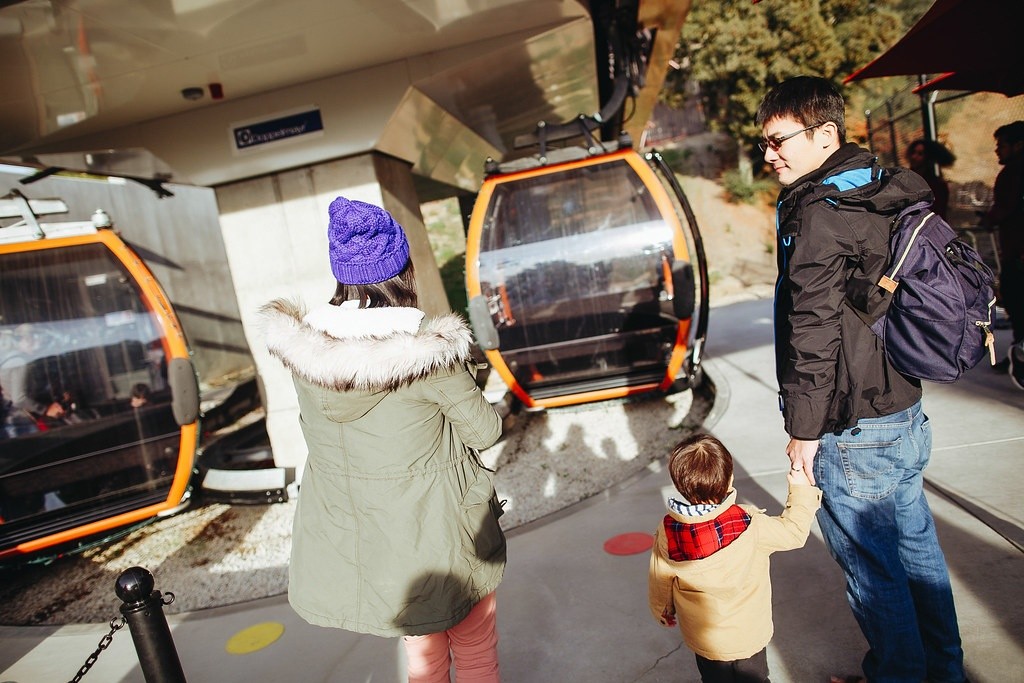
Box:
[328,196,410,284]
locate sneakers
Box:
[1007,345,1024,390]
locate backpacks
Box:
[843,202,996,383]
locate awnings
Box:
[839,0,1024,98]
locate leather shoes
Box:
[991,358,1010,372]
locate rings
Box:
[791,462,803,472]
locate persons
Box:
[261,195,508,683]
[847,122,1024,390]
[754,76,967,682]
[0,322,155,418]
[649,434,823,683]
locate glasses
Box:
[758,122,826,152]
